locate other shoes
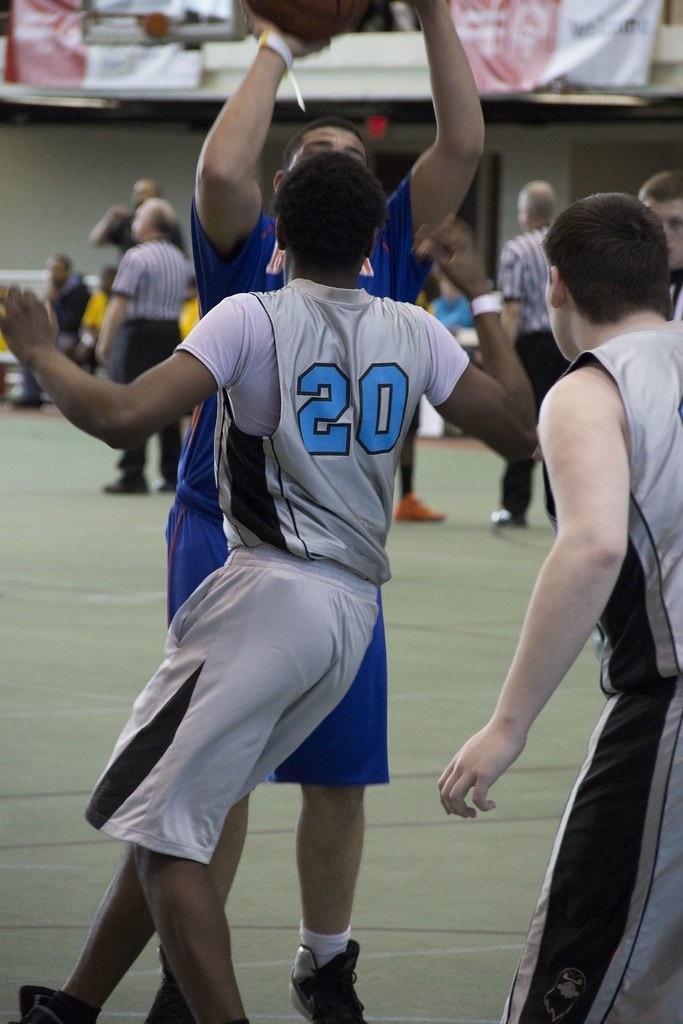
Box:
[106,483,147,492]
[490,509,526,525]
[156,480,177,490]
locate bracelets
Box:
[257,29,293,75]
[470,295,504,319]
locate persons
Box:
[1,172,573,529]
[0,151,540,1023]
[436,193,683,1024]
[638,170,683,424]
[139,1,487,1023]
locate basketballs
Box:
[250,0,370,42]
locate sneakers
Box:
[292,939,369,1024]
[145,945,197,1024]
[395,498,445,520]
